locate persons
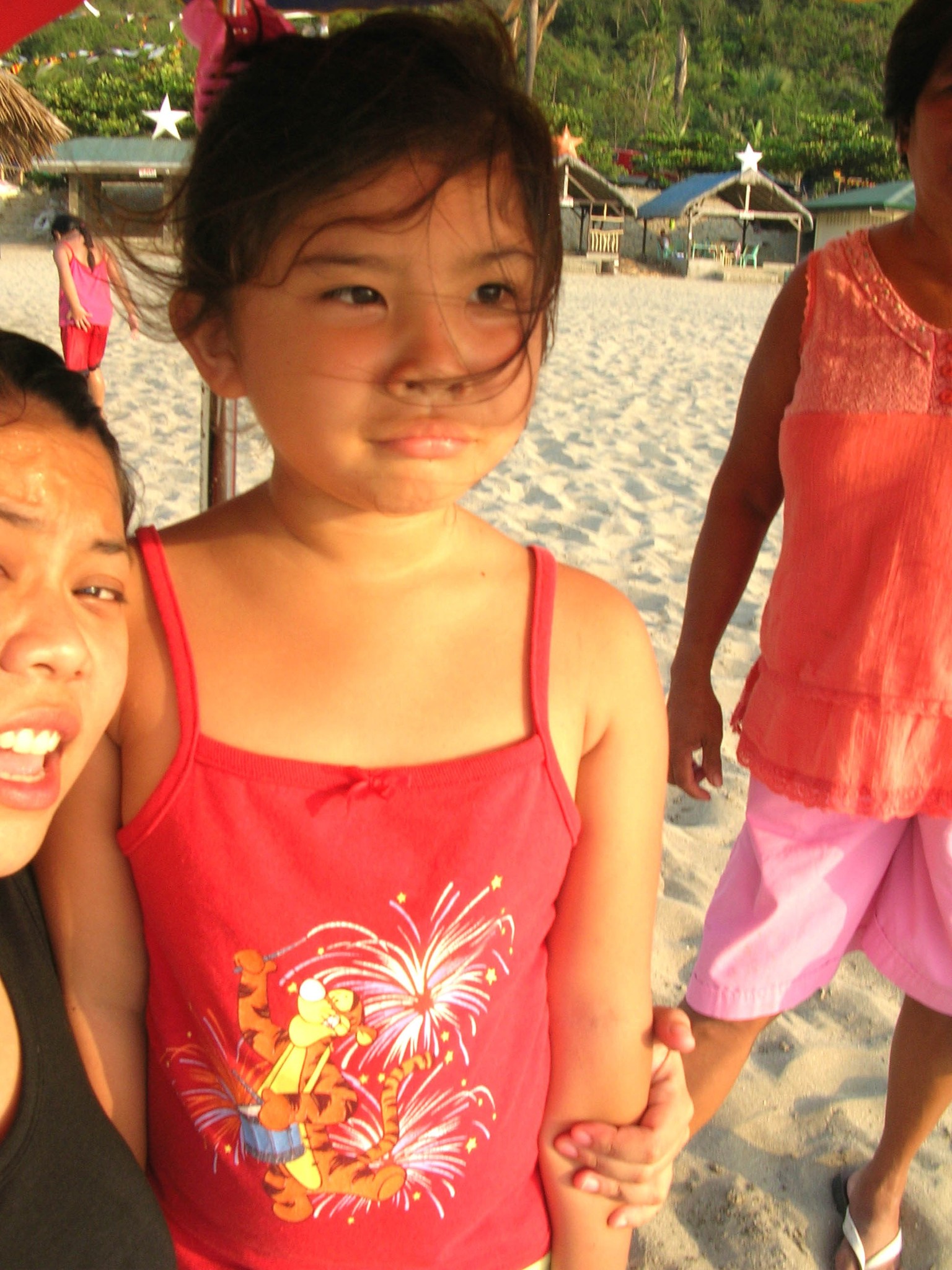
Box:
[657,1,952,1270]
[0,326,705,1270]
[27,11,673,1270]
[50,214,139,425]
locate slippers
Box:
[834,1165,902,1269]
